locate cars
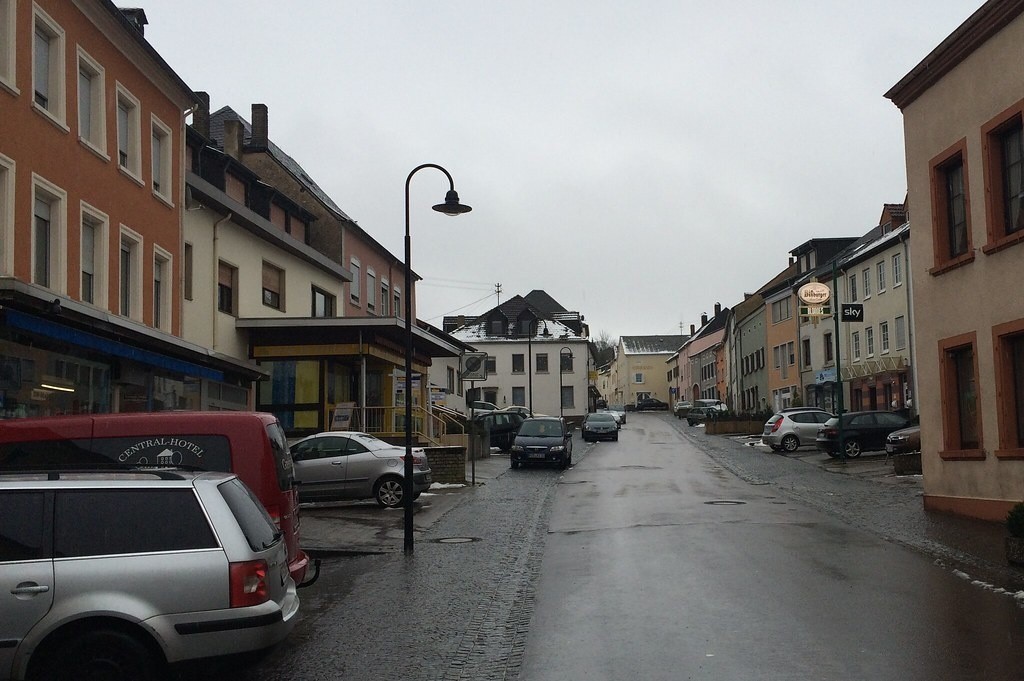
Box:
[287,430,433,508]
[884,425,922,456]
[492,406,551,418]
[607,404,627,424]
[468,401,501,417]
[603,411,621,429]
[816,411,912,461]
[596,400,606,409]
[636,398,669,411]
[473,411,525,450]
[686,406,719,426]
[581,413,618,442]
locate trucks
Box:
[694,399,729,411]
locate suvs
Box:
[510,416,574,471]
[1,470,300,681]
[761,406,836,452]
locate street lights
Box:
[402,161,474,550]
[528,317,554,418]
[559,347,576,416]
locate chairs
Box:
[502,417,509,424]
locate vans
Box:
[0,413,310,586]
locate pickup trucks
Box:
[673,401,693,416]
